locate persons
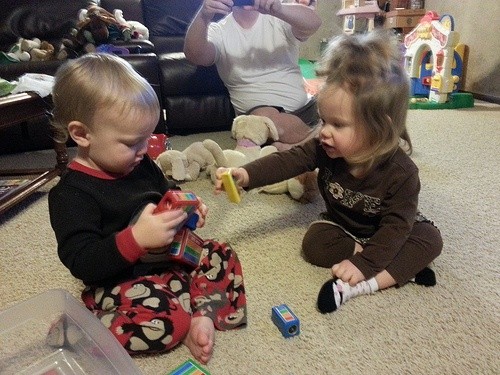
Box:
[182,0,325,183]
[39,50,249,366]
[212,29,444,314]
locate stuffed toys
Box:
[0,0,154,66]
[216,111,306,200]
[153,136,228,187]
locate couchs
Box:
[1,0,234,153]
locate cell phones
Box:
[232,0,255,6]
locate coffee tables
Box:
[0,81,73,214]
